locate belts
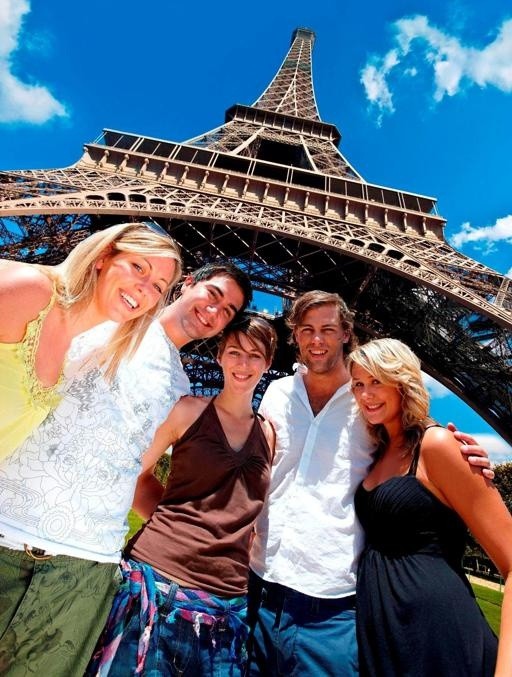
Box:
[0,535,64,559]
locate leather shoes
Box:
[169,604,230,635]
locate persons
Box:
[0,262,255,677]
[0,223,183,461]
[181,290,495,676]
[343,338,512,677]
[84,314,276,677]
